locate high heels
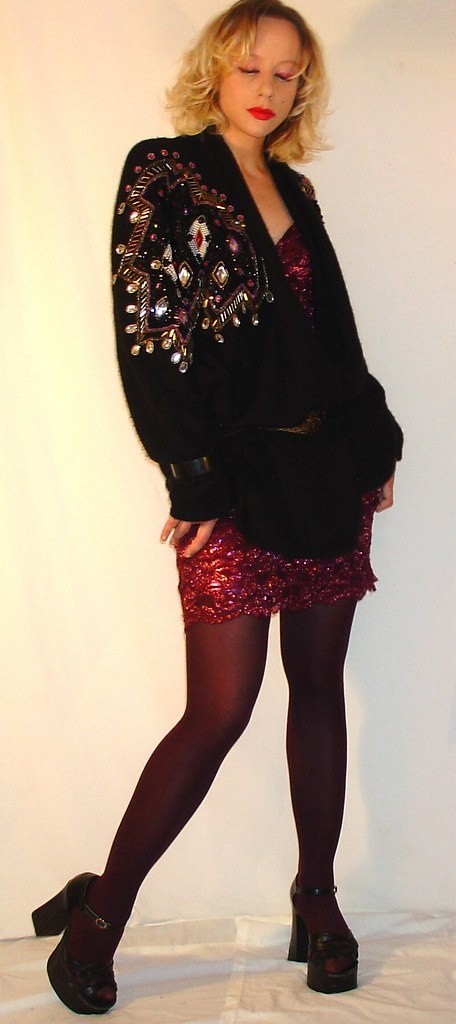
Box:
[31,872,116,1015]
[288,872,359,994]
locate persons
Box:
[32,1,403,1015]
